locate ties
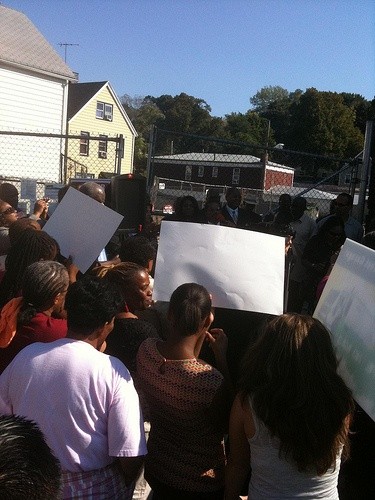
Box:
[233,211,237,225]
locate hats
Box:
[244,194,258,205]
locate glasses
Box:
[3,207,15,216]
[334,201,351,207]
[329,231,344,236]
[207,207,220,211]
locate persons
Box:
[0,174,375,500]
